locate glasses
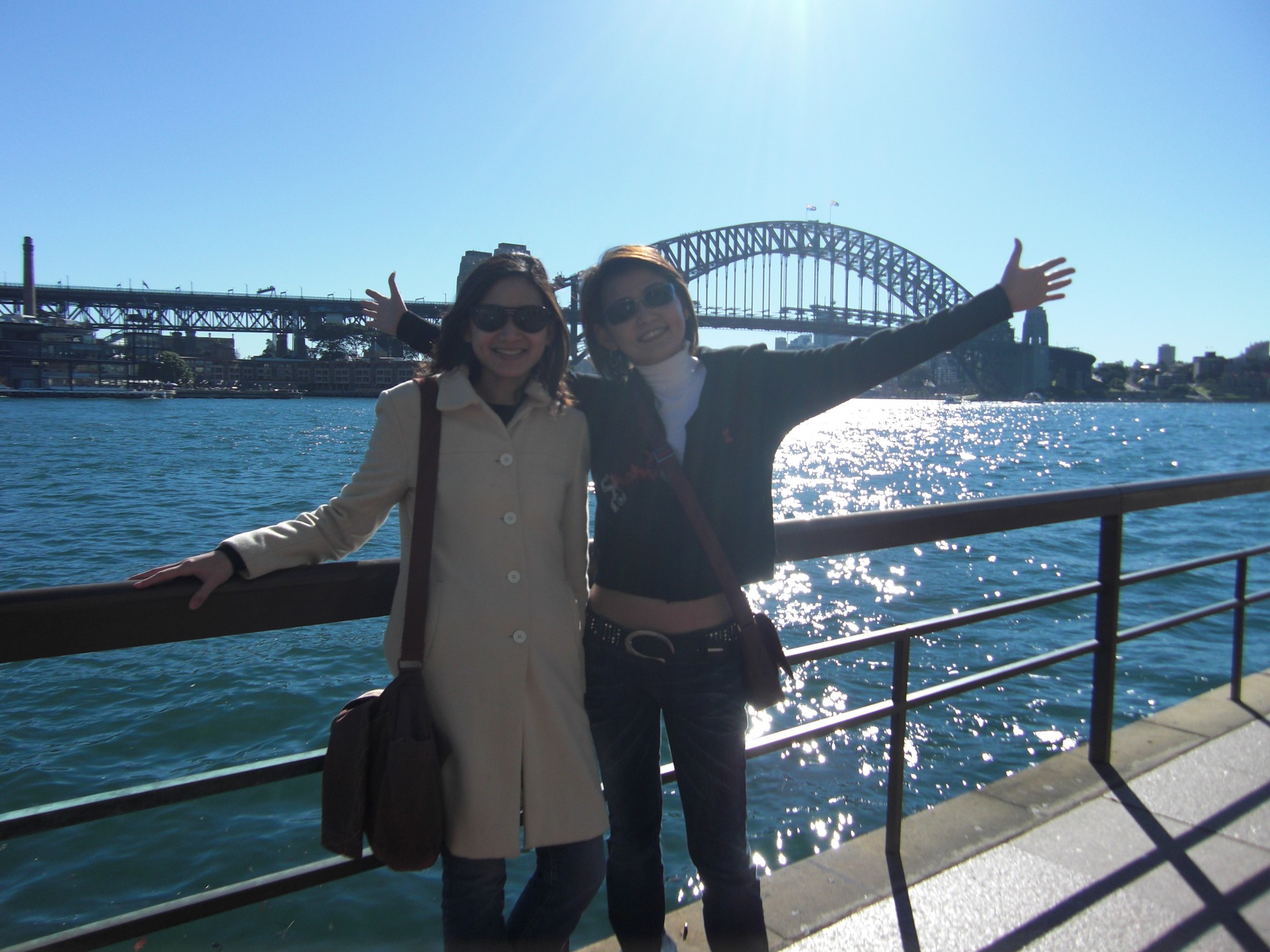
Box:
[594,282,676,326]
[467,301,555,334]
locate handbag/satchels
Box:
[320,676,445,871]
[736,612,794,712]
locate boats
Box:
[943,394,979,404]
[1022,391,1044,403]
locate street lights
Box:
[348,289,351,298]
[190,281,192,291]
[65,275,69,285]
[443,293,446,302]
[4,271,6,283]
[129,278,131,288]
[244,283,248,293]
[300,286,302,296]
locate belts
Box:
[585,612,742,663]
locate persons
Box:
[124,252,610,952]
[362,236,1076,952]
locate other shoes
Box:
[660,933,677,952]
